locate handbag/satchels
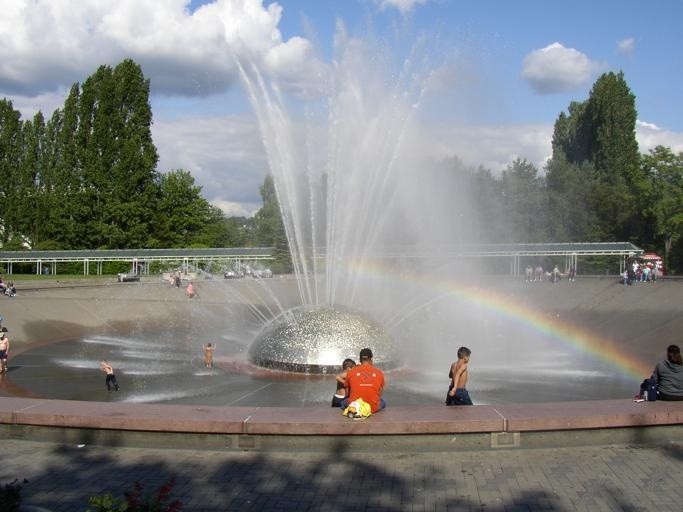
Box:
[342,397,372,420]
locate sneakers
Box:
[0,367,8,373]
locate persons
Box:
[567,267,576,282]
[532,264,543,282]
[330,358,356,408]
[633,344,683,402]
[0,314,3,328]
[444,345,475,407]
[44,266,49,275]
[0,331,9,374]
[337,347,388,414]
[97,359,120,392]
[524,265,533,283]
[621,257,660,287]
[164,269,197,300]
[0,277,17,298]
[119,270,127,283]
[201,342,217,369]
[221,266,272,279]
[550,265,561,283]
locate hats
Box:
[0,331,5,337]
[360,348,373,359]
